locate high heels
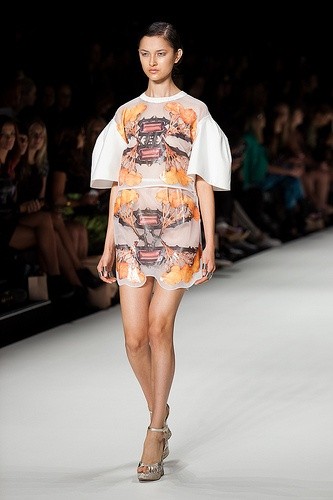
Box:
[157,420,170,459]
[136,422,164,481]
[77,269,104,291]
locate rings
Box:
[206,271,211,277]
[98,271,103,277]
[101,265,108,272]
[202,261,207,270]
[208,274,212,280]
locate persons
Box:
[0,104,333,311]
[95,16,233,483]
